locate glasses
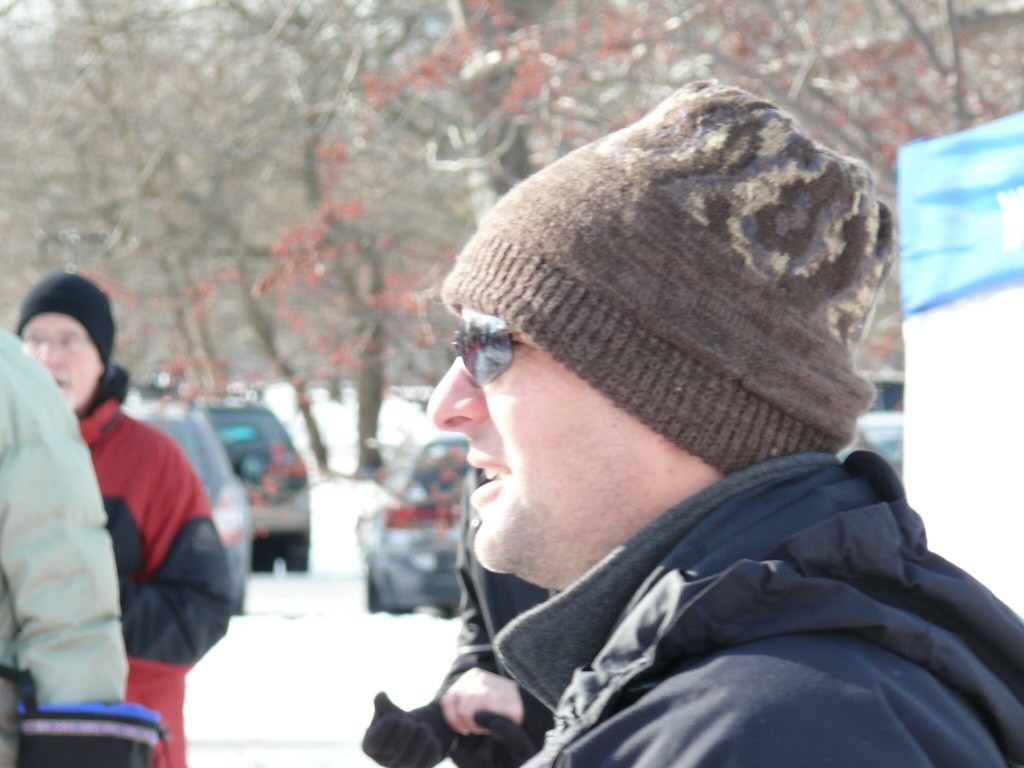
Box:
[450,318,521,388]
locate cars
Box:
[200,403,311,574]
[843,368,907,484]
[361,437,474,621]
[129,402,252,615]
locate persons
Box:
[362,78,1024,768]
[0,270,231,768]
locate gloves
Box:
[362,691,456,768]
[450,715,535,768]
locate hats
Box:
[17,274,114,370]
[441,80,895,475]
